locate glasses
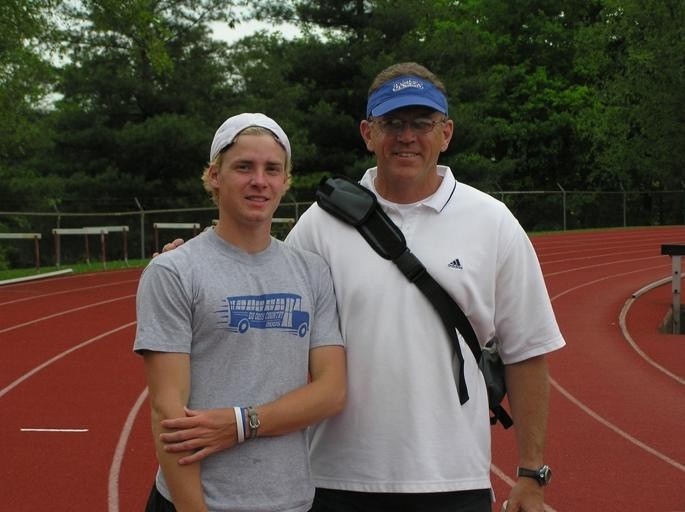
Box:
[368,116,446,134]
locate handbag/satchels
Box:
[474,342,509,406]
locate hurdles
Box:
[0,219,294,272]
[658,243,684,333]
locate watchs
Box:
[516,465,552,487]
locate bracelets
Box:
[243,406,260,442]
[233,406,247,444]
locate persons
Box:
[152,61,566,512]
[132,111,347,512]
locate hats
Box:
[365,75,449,119]
[210,112,292,163]
